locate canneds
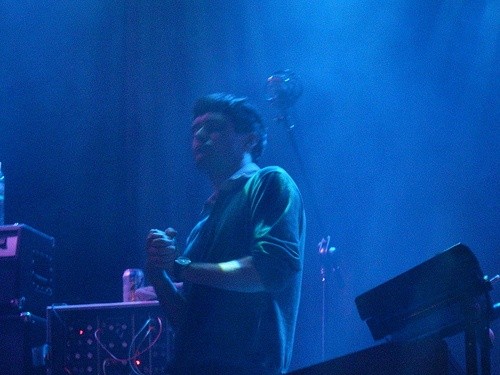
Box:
[123,269,145,301]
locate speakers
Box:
[288,337,465,375]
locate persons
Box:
[146,93,306,375]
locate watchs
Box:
[173,255,192,281]
